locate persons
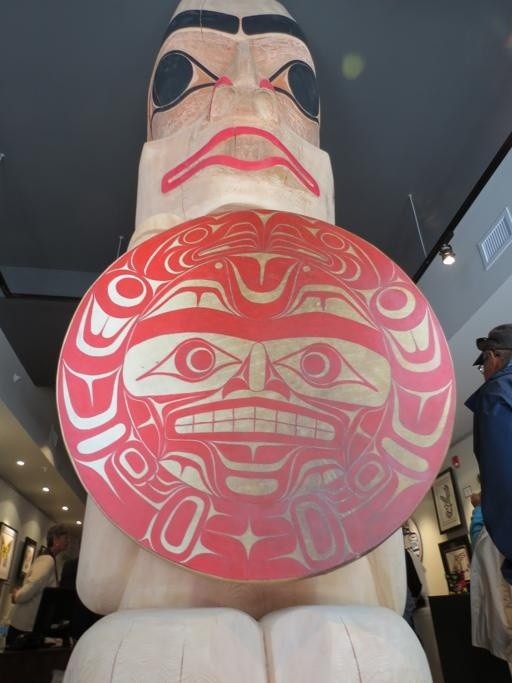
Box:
[5,525,70,644]
[470,493,512,678]
[464,323,512,585]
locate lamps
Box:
[439,242,456,264]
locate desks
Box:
[428,590,512,683]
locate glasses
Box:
[478,351,494,375]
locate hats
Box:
[471,323,512,366]
[47,525,70,538]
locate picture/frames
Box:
[402,517,423,563]
[430,466,463,535]
[438,534,472,591]
[0,521,19,582]
[18,536,37,580]
